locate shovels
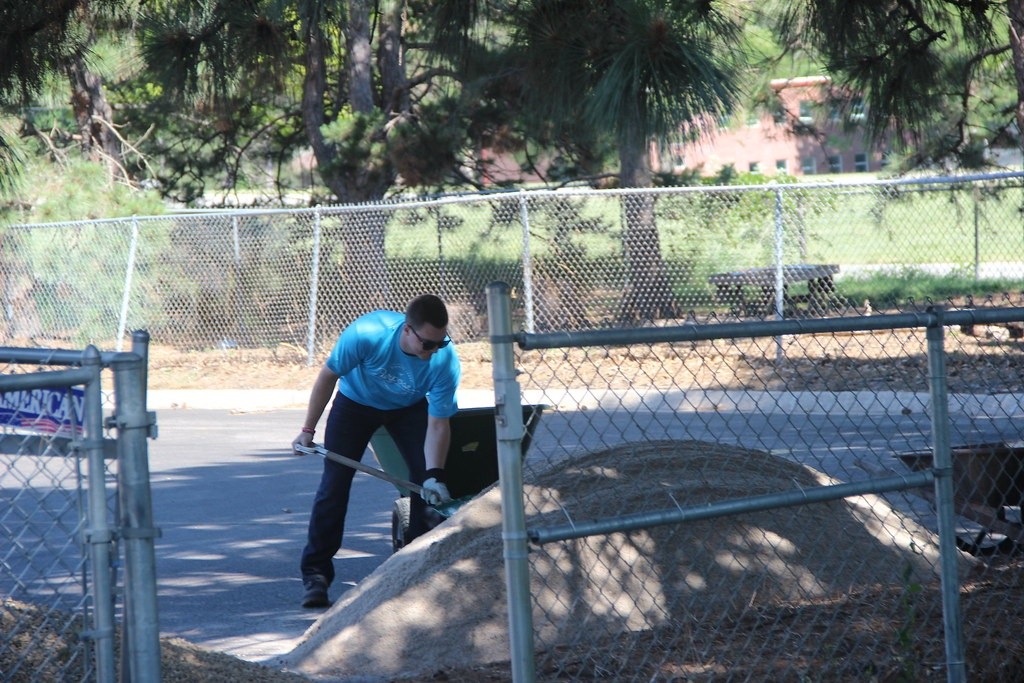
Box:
[294,441,475,517]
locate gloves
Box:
[420,468,451,506]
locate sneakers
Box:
[301,583,329,607]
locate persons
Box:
[291,295,459,606]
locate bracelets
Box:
[422,468,445,483]
[302,427,315,435]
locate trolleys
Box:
[369,401,553,555]
[890,441,1024,557]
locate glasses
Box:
[407,325,451,350]
